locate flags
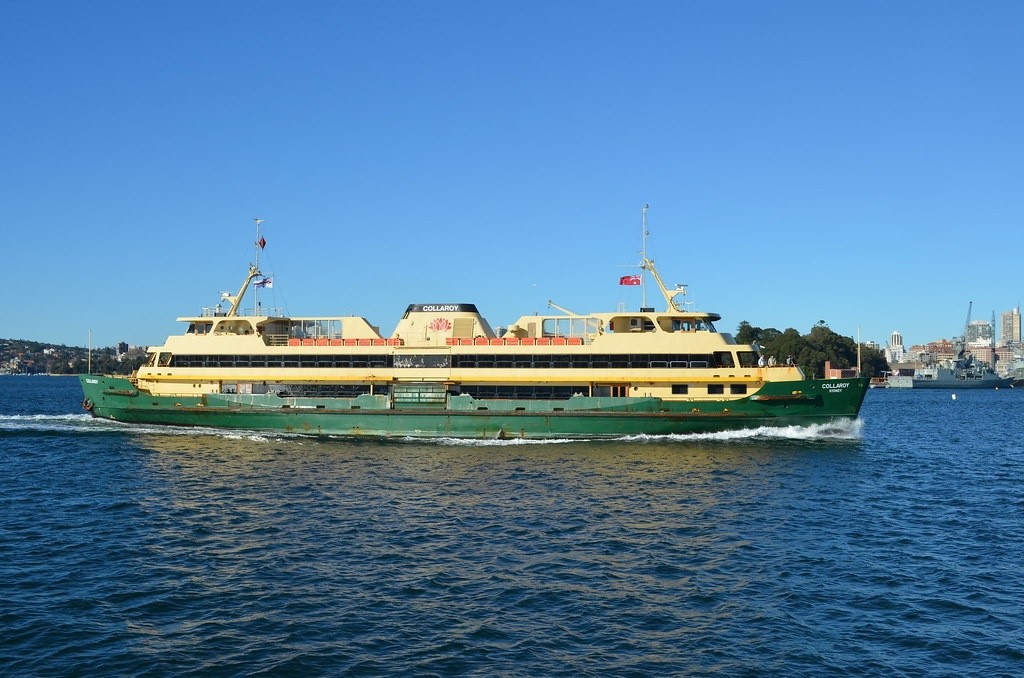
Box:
[619,275,640,285]
[260,236,266,249]
[257,272,274,288]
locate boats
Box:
[887,348,1015,389]
[76,200,872,440]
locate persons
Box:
[768,355,776,367]
[758,355,767,368]
[787,355,796,366]
